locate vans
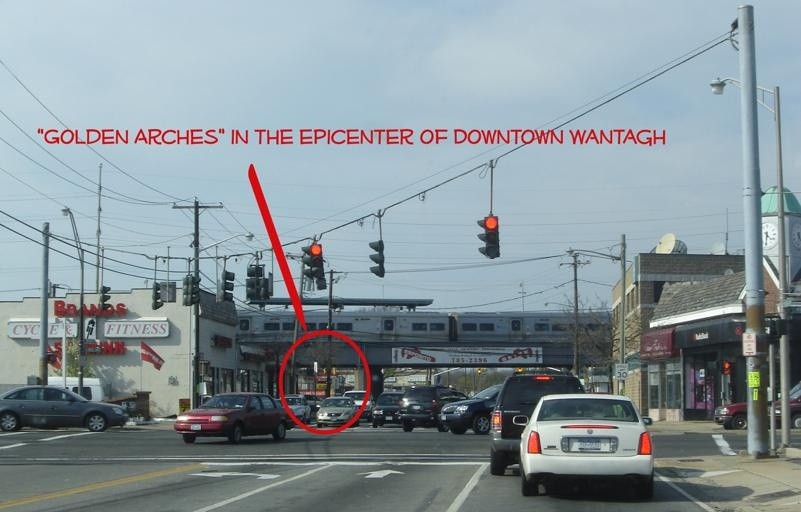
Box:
[27,377,106,402]
[485,369,587,476]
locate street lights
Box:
[710,76,792,448]
[190,231,255,410]
[60,205,84,398]
[566,246,626,396]
[544,302,578,377]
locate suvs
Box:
[400,386,471,433]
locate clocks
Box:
[761,222,778,250]
[791,221,801,252]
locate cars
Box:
[513,392,655,496]
[0,385,130,432]
[440,384,507,434]
[279,389,407,429]
[715,376,801,430]
[174,392,295,443]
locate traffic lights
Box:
[222,271,235,302]
[152,282,163,310]
[516,367,526,373]
[484,215,501,258]
[183,277,202,306]
[721,358,737,375]
[369,240,385,277]
[477,367,482,376]
[301,243,328,291]
[245,264,274,303]
[478,220,495,260]
[99,286,111,310]
[47,352,57,362]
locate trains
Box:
[235,310,612,343]
